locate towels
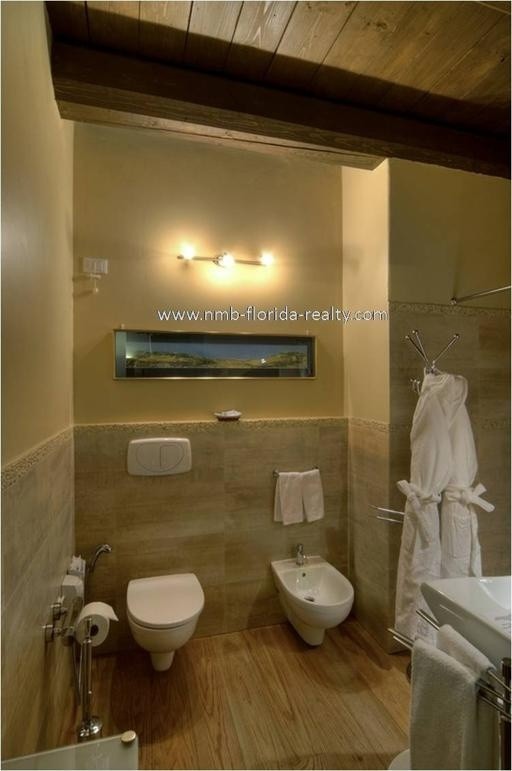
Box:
[274,469,326,525]
[409,623,502,768]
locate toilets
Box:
[127,573,205,673]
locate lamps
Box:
[178,243,274,270]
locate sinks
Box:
[270,555,354,647]
[419,577,511,693]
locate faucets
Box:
[296,545,304,564]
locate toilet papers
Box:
[76,601,119,647]
